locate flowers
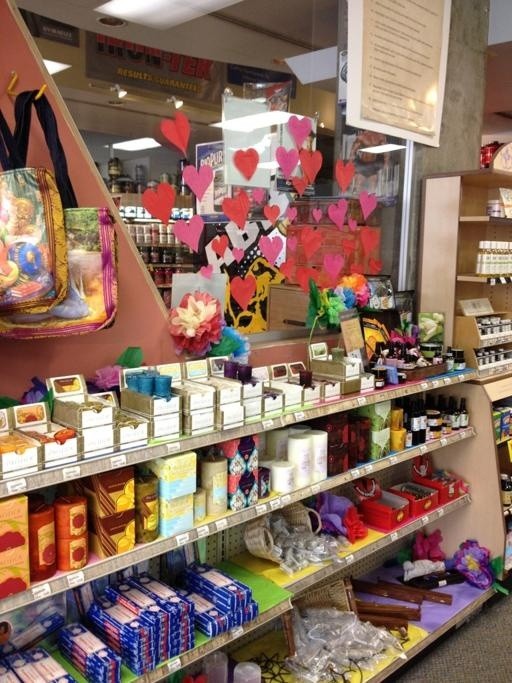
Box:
[307,273,371,330]
[168,290,223,352]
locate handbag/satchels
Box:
[0,166,116,342]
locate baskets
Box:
[279,576,361,654]
[244,504,323,561]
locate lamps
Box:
[109,83,184,109]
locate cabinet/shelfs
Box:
[0,332,509,682]
[414,174,512,383]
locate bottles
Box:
[501,473,512,507]
